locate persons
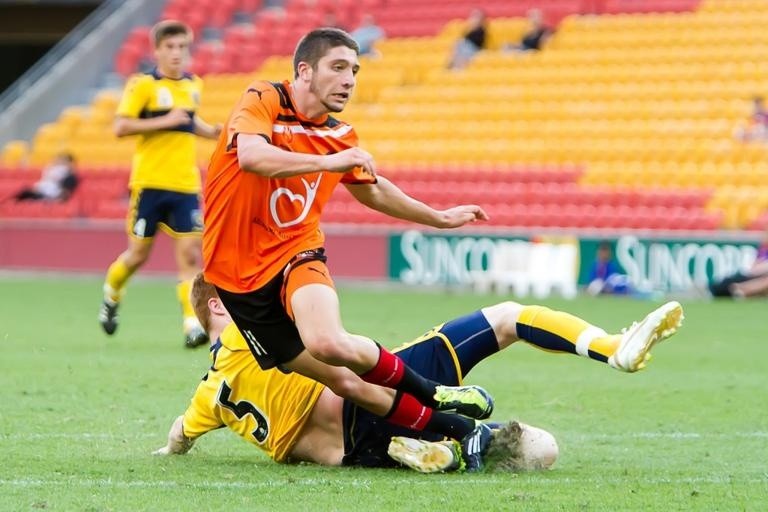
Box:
[197,24,498,461]
[500,6,557,59]
[439,8,488,75]
[346,14,385,56]
[726,232,768,299]
[9,152,78,204]
[730,96,768,147]
[148,264,687,475]
[95,20,228,349]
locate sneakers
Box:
[462,425,492,474]
[607,302,685,373]
[99,298,120,334]
[433,383,494,420]
[185,330,209,347]
[384,435,460,473]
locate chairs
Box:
[0,0,768,248]
